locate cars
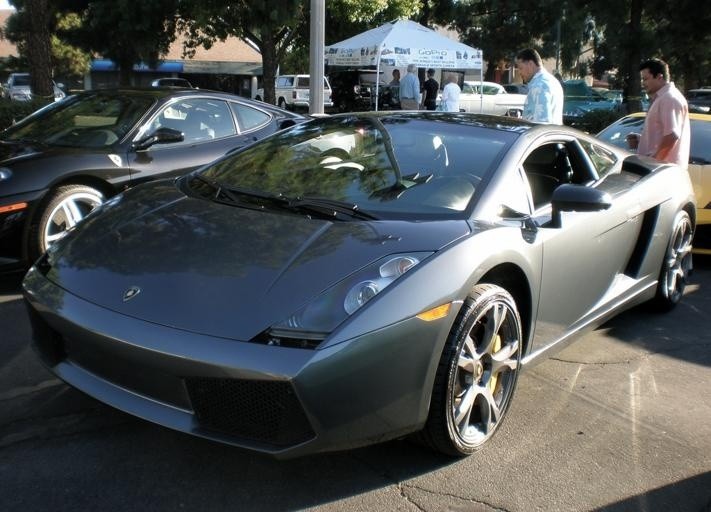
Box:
[424,80,526,118]
[1,73,66,112]
[0,88,319,288]
[147,77,199,94]
[506,76,710,122]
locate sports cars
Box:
[16,110,699,459]
[584,106,710,263]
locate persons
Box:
[512,48,564,124]
[398,64,420,110]
[439,72,461,112]
[389,69,401,86]
[420,68,439,111]
[626,58,690,172]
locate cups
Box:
[627,132,638,152]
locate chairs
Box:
[524,147,560,209]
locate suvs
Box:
[332,68,389,109]
[253,75,337,111]
